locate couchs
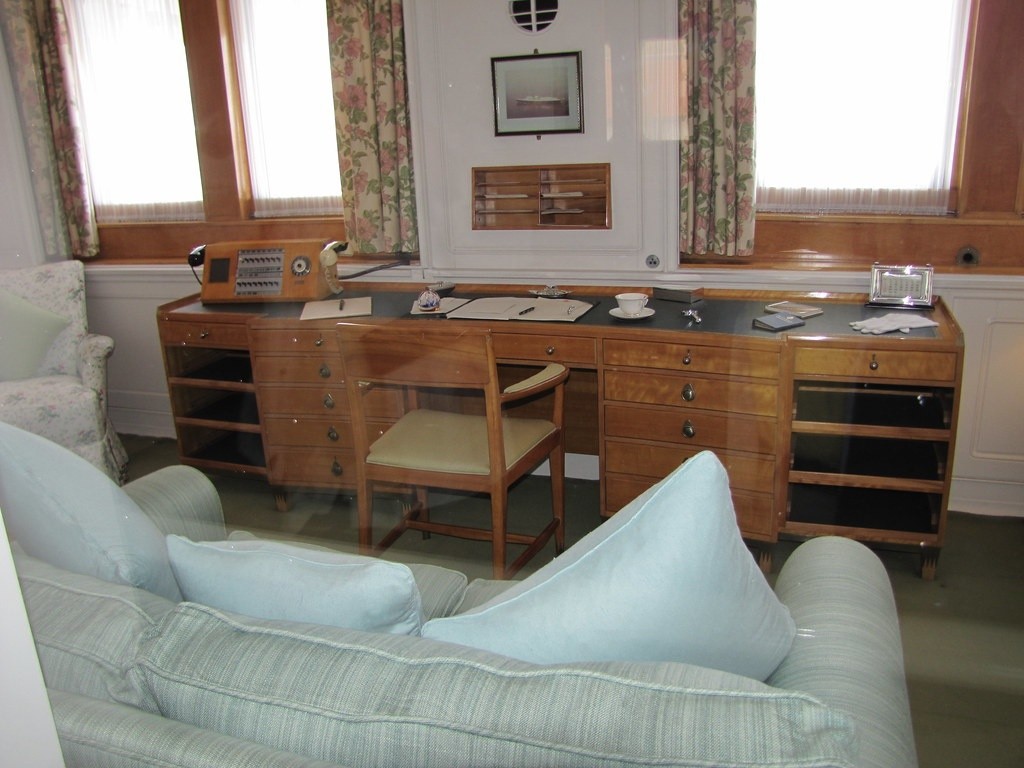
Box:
[0,261,131,490]
[13,454,918,768]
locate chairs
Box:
[335,322,564,582]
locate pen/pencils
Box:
[519,307,535,315]
[340,300,345,310]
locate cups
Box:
[614,293,649,315]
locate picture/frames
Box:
[490,48,585,136]
[870,265,934,307]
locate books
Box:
[752,300,823,332]
[300,296,372,320]
[446,296,600,323]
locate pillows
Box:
[163,530,422,642]
[0,286,71,380]
[422,447,803,689]
[1,422,195,605]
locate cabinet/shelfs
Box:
[470,163,613,230]
[152,261,967,583]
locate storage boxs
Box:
[651,284,704,303]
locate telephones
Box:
[188,237,350,303]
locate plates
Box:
[608,307,655,319]
